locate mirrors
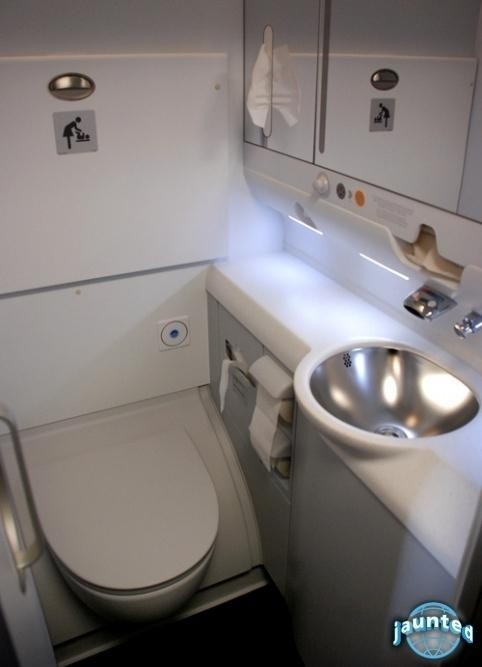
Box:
[243,0,481,226]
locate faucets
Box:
[402,285,455,321]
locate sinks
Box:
[293,342,479,461]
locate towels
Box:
[246,44,303,129]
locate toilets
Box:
[0,386,269,667]
